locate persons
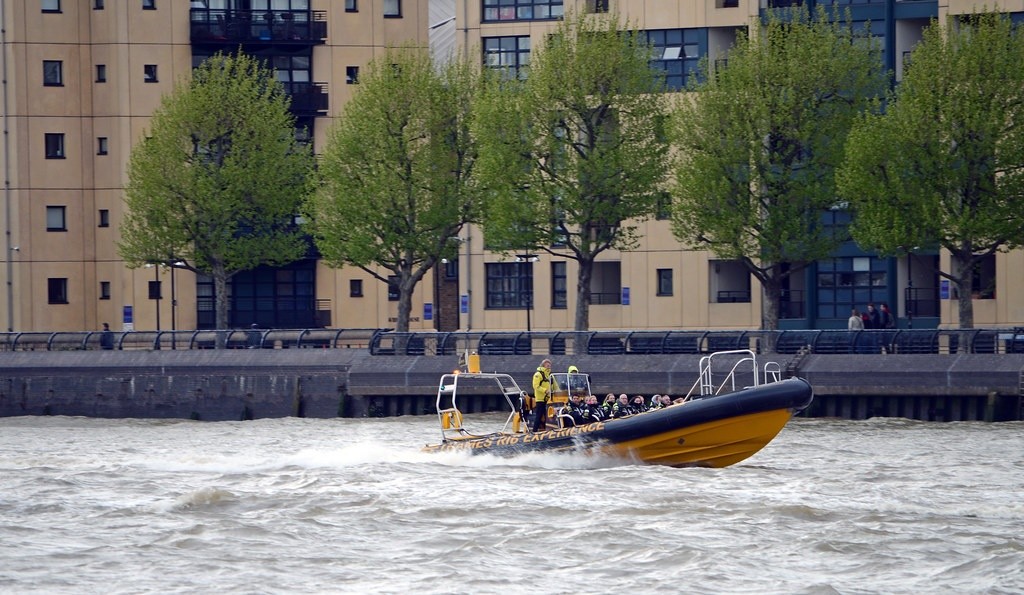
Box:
[881,302,896,354]
[533,359,561,432]
[650,393,672,413]
[559,365,592,390]
[630,396,648,416]
[860,311,871,328]
[610,394,635,418]
[247,323,262,349]
[602,390,616,420]
[868,305,881,329]
[848,308,865,330]
[563,394,600,428]
[99,323,115,351]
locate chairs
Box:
[558,407,578,429]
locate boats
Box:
[429,346,817,474]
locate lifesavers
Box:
[441,407,464,430]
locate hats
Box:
[540,359,552,367]
[568,365,579,374]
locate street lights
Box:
[516,254,543,336]
[146,260,189,352]
[896,244,924,348]
[434,258,454,333]
